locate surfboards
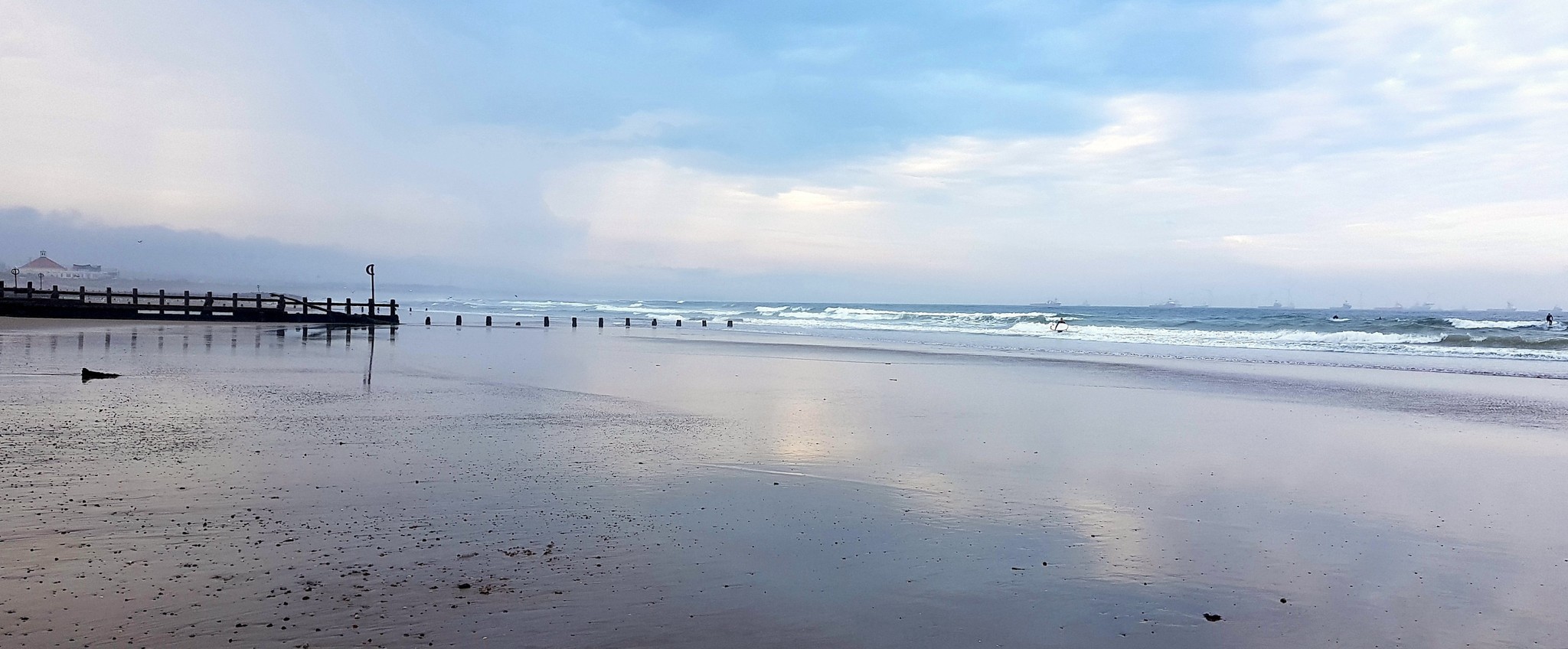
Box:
[1547,325,1554,327]
[1050,323,1068,330]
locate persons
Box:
[1055,318,1066,332]
[1546,313,1553,325]
[1333,315,1337,319]
[1379,317,1382,320]
